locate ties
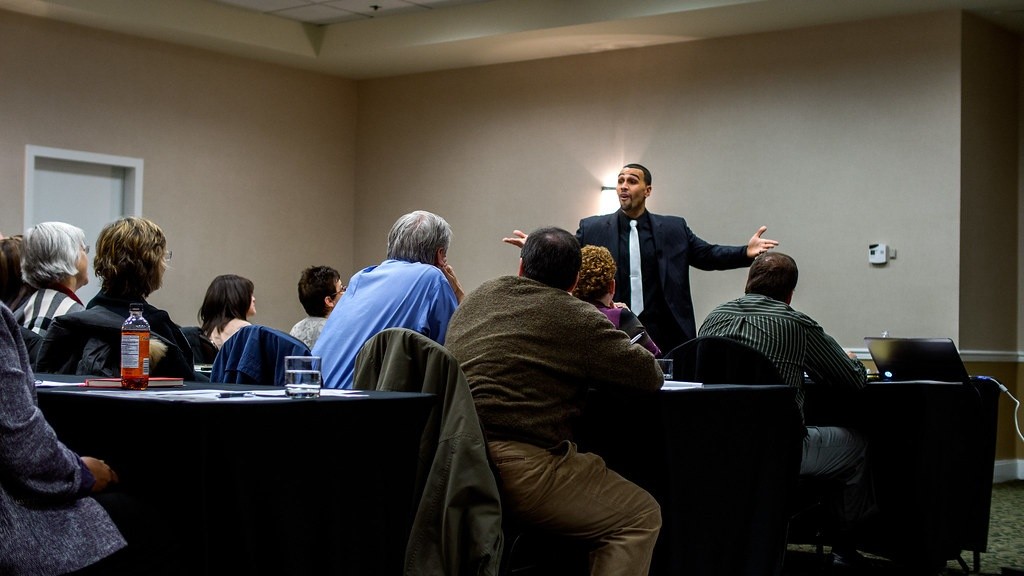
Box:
[629,220,644,317]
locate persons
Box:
[197,274,257,351]
[0,234,38,313]
[86,216,210,383]
[289,265,347,351]
[571,244,662,365]
[12,221,91,337]
[443,227,666,576]
[0,301,192,576]
[311,210,465,390]
[698,252,868,566]
[502,164,779,359]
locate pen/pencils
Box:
[215,393,244,398]
[631,332,644,344]
[201,367,212,371]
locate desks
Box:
[649,379,791,576]
[804,377,999,576]
[35,372,442,576]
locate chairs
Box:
[662,337,824,576]
[355,327,598,576]
[215,325,312,386]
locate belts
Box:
[485,429,555,452]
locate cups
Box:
[284,355,322,398]
[653,358,674,380]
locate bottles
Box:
[120,302,150,390]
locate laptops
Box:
[865,336,994,382]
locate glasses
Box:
[163,250,172,262]
[331,286,347,296]
